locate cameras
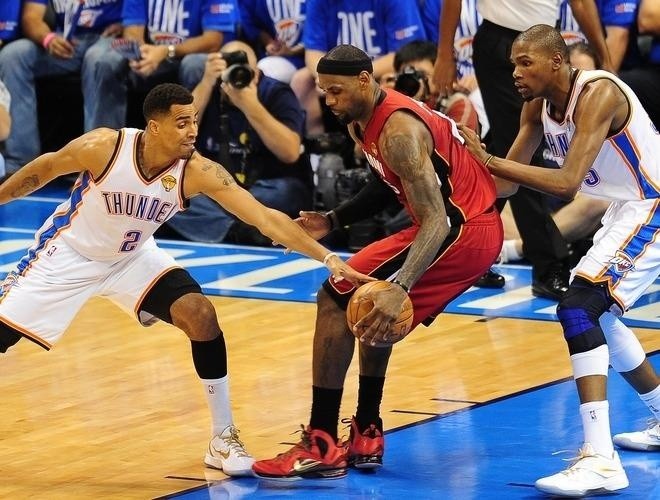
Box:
[221,50,256,90]
[392,65,429,98]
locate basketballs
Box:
[346,281,413,347]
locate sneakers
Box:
[613,423,660,451]
[534,443,629,497]
[343,416,386,471]
[204,424,256,475]
[252,425,351,485]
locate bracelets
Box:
[392,279,411,293]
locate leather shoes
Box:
[531,280,570,299]
[472,271,506,290]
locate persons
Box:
[521,0,642,186]
[549,41,633,245]
[463,23,660,496]
[431,0,621,300]
[290,3,428,145]
[156,40,313,246]
[253,45,504,483]
[398,45,482,148]
[0,83,376,481]
[229,0,306,137]
[117,1,240,148]
[0,0,143,183]
